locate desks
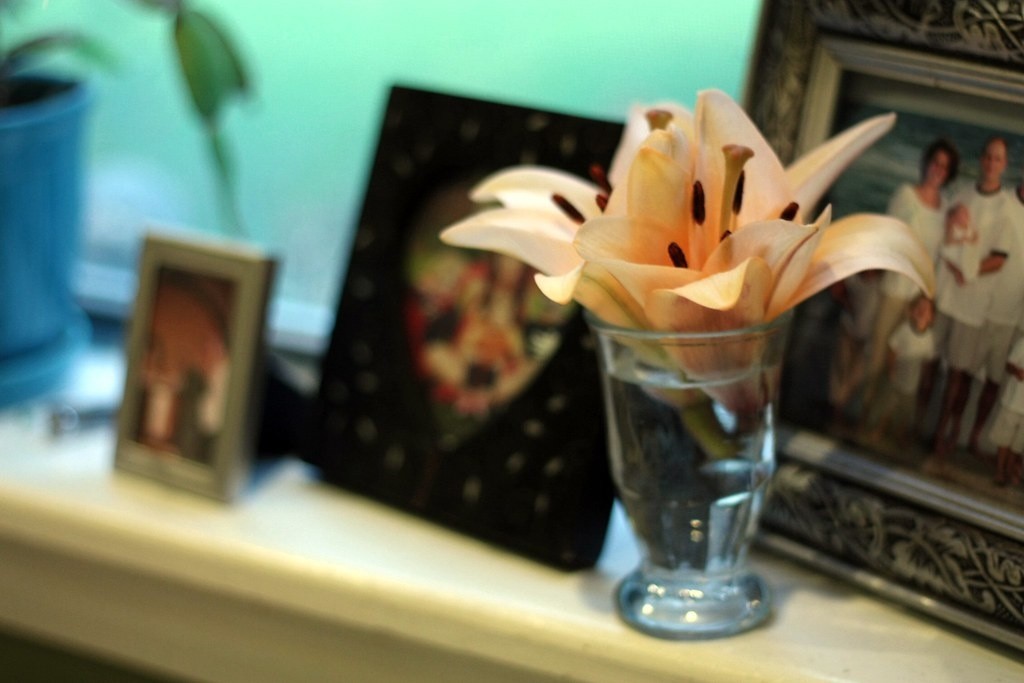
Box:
[0,348,1024,682]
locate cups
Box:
[591,302,782,634]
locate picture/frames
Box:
[700,3,1024,656]
[113,234,278,505]
[307,84,625,573]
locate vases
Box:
[580,311,794,634]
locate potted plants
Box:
[0,0,263,411]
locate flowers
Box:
[441,92,940,466]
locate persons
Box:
[982,337,1024,484]
[145,334,232,460]
[414,252,543,405]
[825,268,885,404]
[851,140,958,407]
[938,204,982,286]
[944,177,1023,451]
[876,294,938,444]
[911,138,1009,450]
